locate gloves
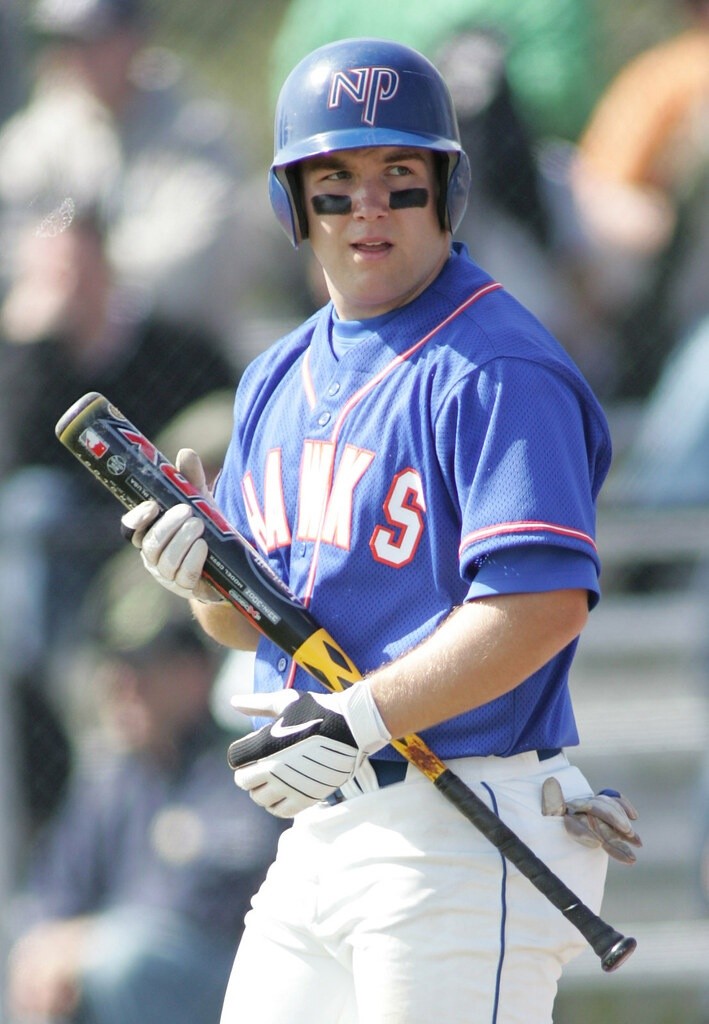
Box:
[226,679,393,819]
[542,775,644,866]
[120,448,249,605]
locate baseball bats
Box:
[54,390,637,973]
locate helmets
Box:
[268,37,472,251]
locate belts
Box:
[315,746,562,809]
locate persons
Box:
[0,0,709,1024]
